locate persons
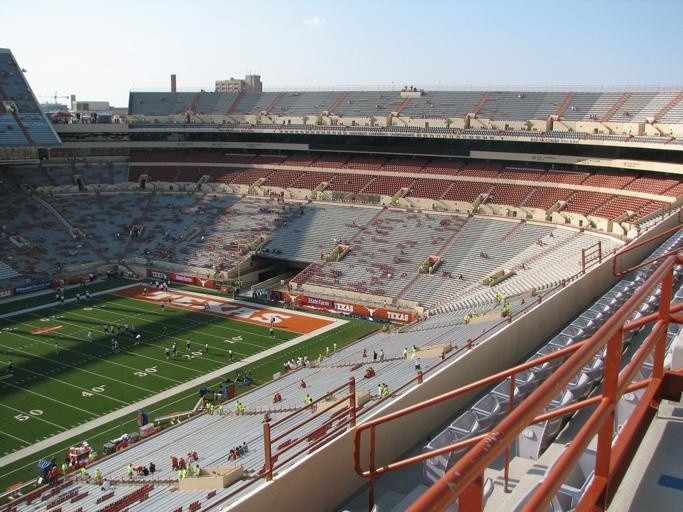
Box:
[50,313,507,413]
[5,359,15,373]
[1,188,527,312]
[45,411,271,491]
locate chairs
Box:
[425,227,683,512]
